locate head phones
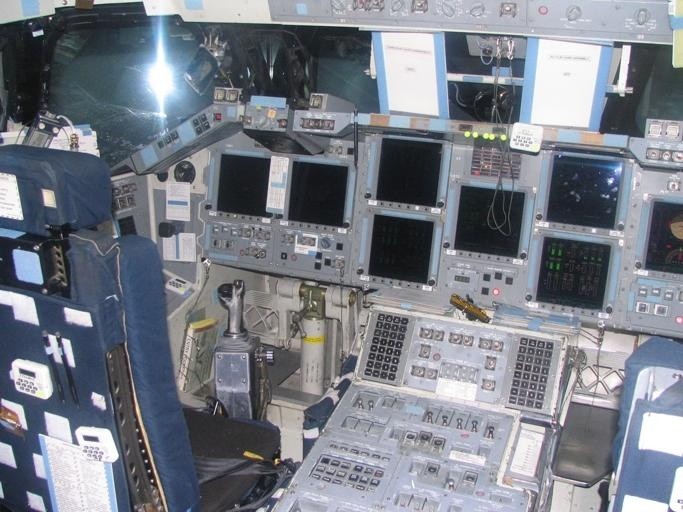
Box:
[454,79,513,124]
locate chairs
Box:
[0,143,280,509]
